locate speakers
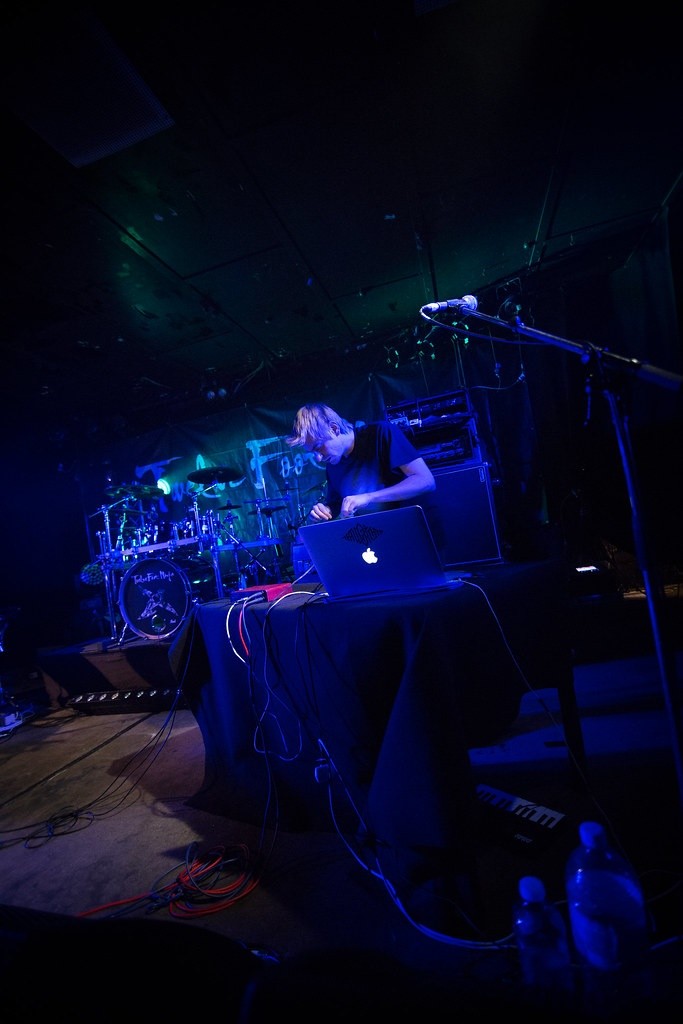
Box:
[396,461,504,567]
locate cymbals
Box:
[105,487,164,497]
[186,467,241,485]
[247,506,287,516]
[217,504,242,511]
[245,499,276,503]
[170,549,219,605]
[275,486,299,491]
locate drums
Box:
[116,558,192,641]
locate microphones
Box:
[422,294,478,313]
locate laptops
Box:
[298,505,479,599]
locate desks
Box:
[188,559,558,824]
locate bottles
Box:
[567,822,649,975]
[516,874,574,985]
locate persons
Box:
[285,402,436,523]
